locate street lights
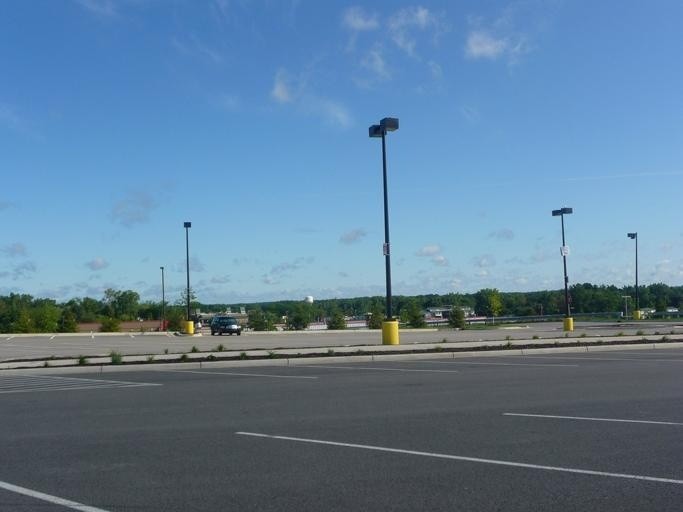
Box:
[551,206,575,332]
[183,220,194,334]
[627,232,641,320]
[367,115,402,344]
[160,266,167,331]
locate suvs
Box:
[210,315,242,336]
[652,306,680,319]
